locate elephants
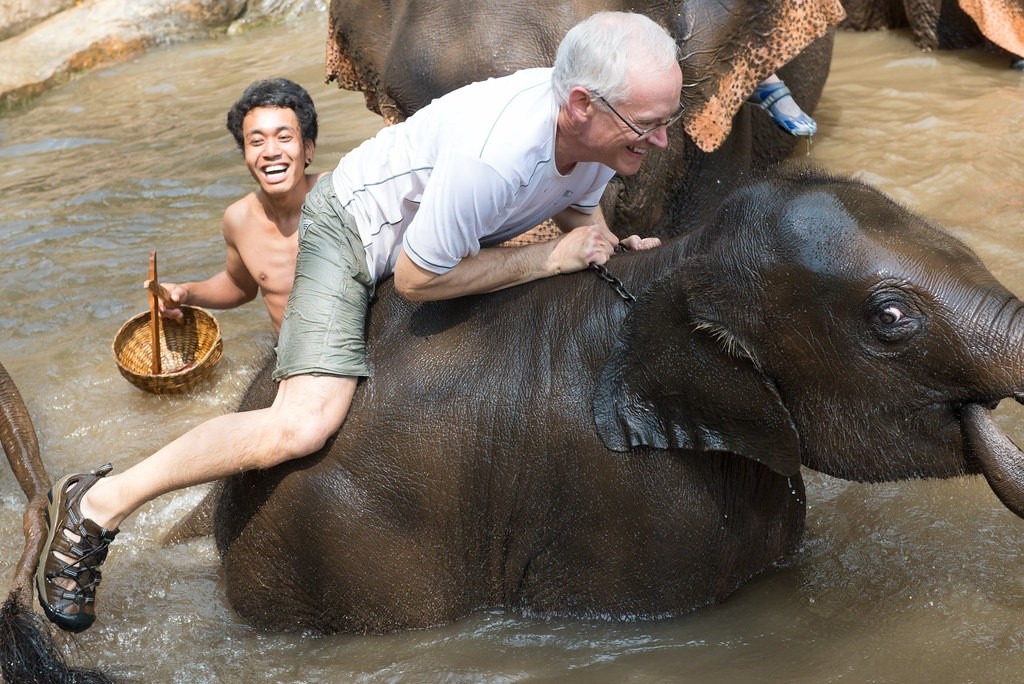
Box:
[0,0,1023,684]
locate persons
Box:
[34,10,691,633]
[152,76,329,337]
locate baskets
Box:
[111,252,224,398]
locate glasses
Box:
[586,89,686,143]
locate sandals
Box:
[34,463,120,631]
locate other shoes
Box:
[746,80,818,138]
